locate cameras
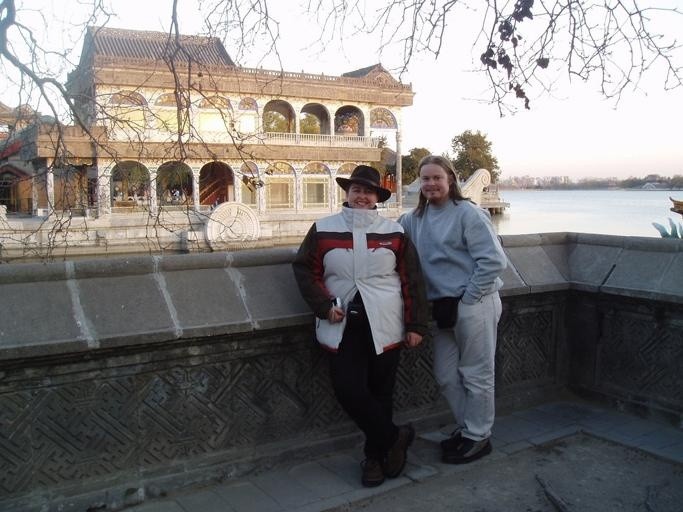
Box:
[331,297,342,309]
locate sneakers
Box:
[361,447,385,488]
[440,434,494,465]
[437,429,464,451]
[381,423,415,479]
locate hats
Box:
[335,164,392,204]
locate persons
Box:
[292,165,429,488]
[170,188,180,201]
[397,153,509,467]
